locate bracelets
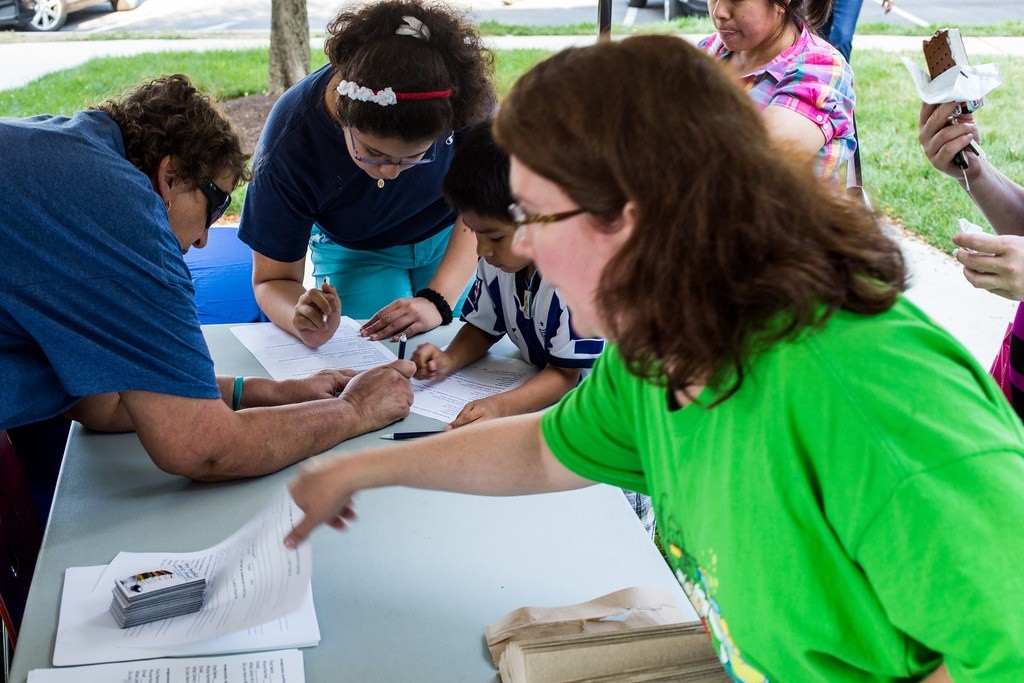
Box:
[232,375,244,412]
[414,287,454,326]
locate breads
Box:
[922,27,972,81]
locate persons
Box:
[282,31,1024,681]
[700,0,857,193]
[918,95,1024,429]
[407,119,656,534]
[815,0,894,64]
[0,71,417,483]
[238,0,503,351]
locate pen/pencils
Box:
[398,333,409,361]
[320,275,332,323]
[378,429,446,441]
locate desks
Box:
[5,318,732,682]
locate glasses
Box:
[348,123,438,167]
[188,166,232,227]
[507,200,592,241]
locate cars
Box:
[0,0,138,33]
